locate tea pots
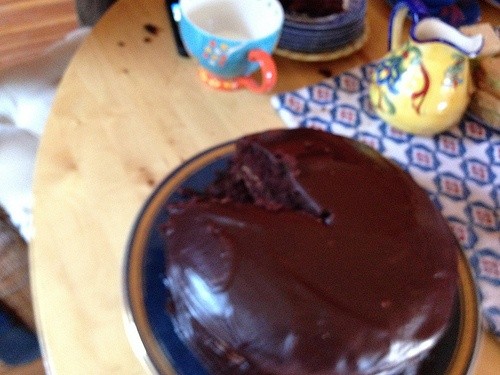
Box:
[368,0,484,136]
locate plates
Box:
[121,130,484,375]
[273,17,370,63]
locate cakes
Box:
[159,127,457,375]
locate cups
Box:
[172,0,284,95]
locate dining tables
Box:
[28,0,500,375]
